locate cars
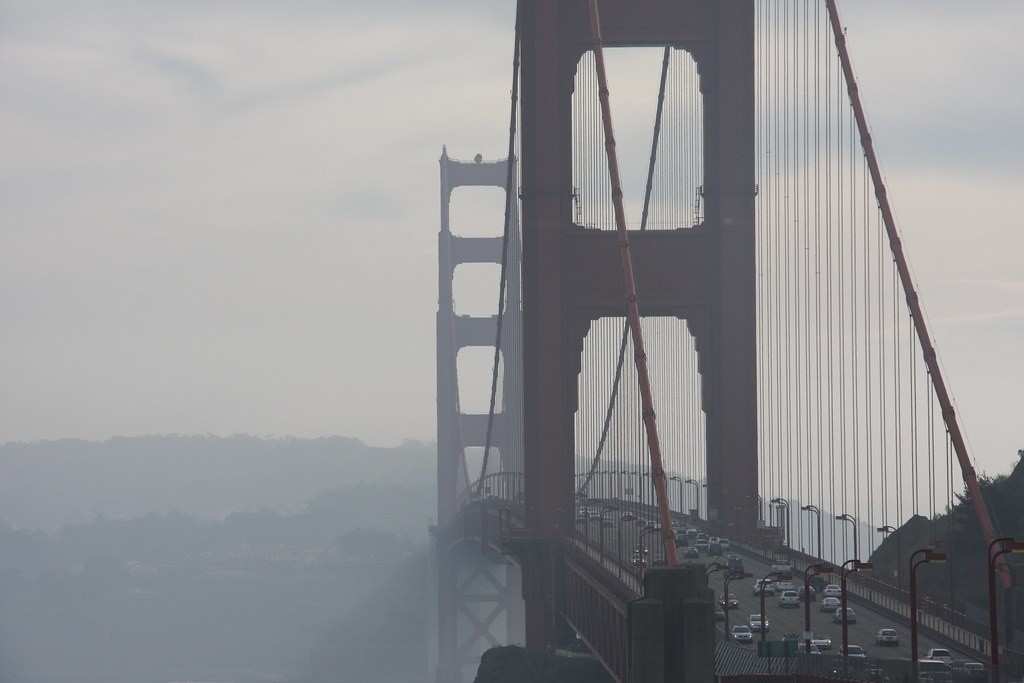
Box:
[776,577,793,591]
[732,625,755,643]
[720,592,738,609]
[748,614,770,632]
[834,605,857,624]
[799,585,819,602]
[771,560,796,576]
[777,588,801,606]
[820,598,843,612]
[928,647,953,664]
[753,578,774,597]
[877,629,899,645]
[800,642,821,655]
[841,645,866,656]
[575,498,742,572]
[822,583,841,596]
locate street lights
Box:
[838,555,872,678]
[585,469,701,591]
[802,555,835,658]
[757,568,790,639]
[878,524,904,603]
[837,513,861,577]
[802,503,825,578]
[770,498,792,560]
[986,536,1022,679]
[904,542,946,681]
[726,572,754,640]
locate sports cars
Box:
[813,632,834,648]
